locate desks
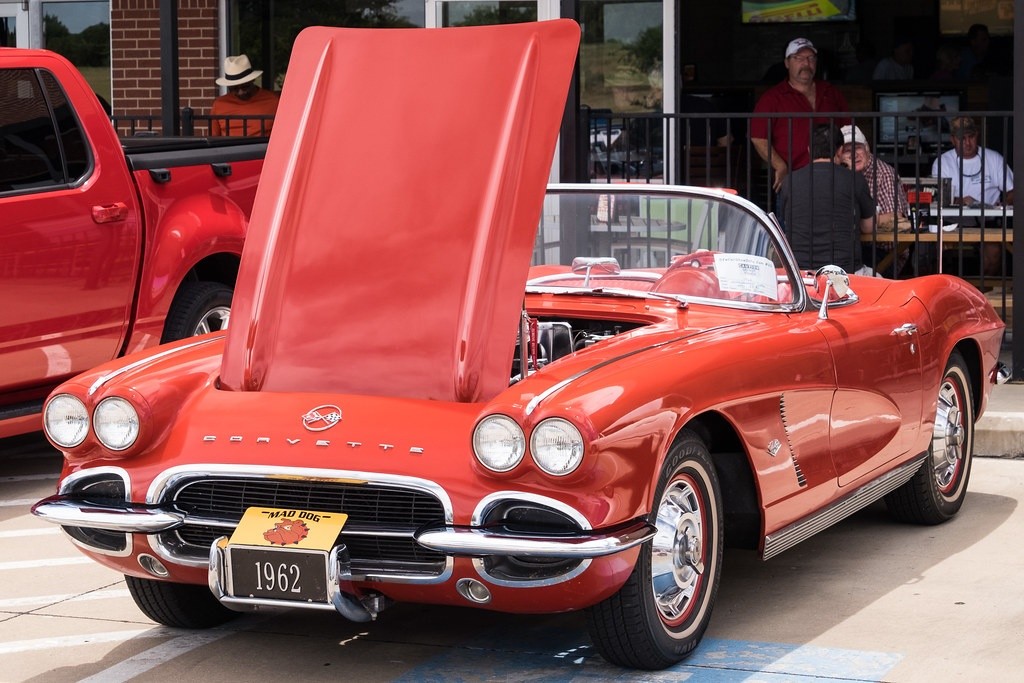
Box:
[928,208,1014,217]
[860,228,1013,272]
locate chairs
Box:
[683,142,744,188]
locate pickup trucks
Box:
[0,48,279,441]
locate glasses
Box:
[791,54,818,63]
[229,82,252,92]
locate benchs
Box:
[550,267,833,309]
[984,293,1013,308]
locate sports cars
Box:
[33,13,1007,672]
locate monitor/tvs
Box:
[873,91,963,150]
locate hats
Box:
[216,55,263,87]
[840,124,867,147]
[786,38,817,58]
[950,115,978,138]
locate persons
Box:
[208,55,279,137]
[749,38,1014,277]
[595,63,718,178]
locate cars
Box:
[584,113,663,178]
